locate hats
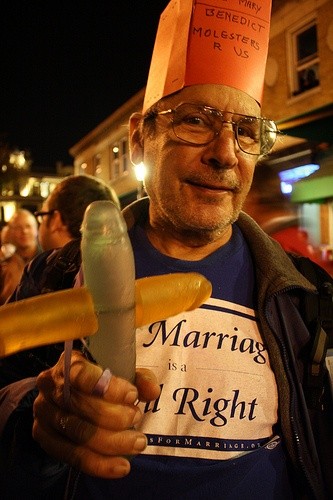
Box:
[141,0,272,117]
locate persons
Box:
[1,175,122,388]
[1,0,333,499]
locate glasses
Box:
[144,101,280,156]
[35,210,55,224]
